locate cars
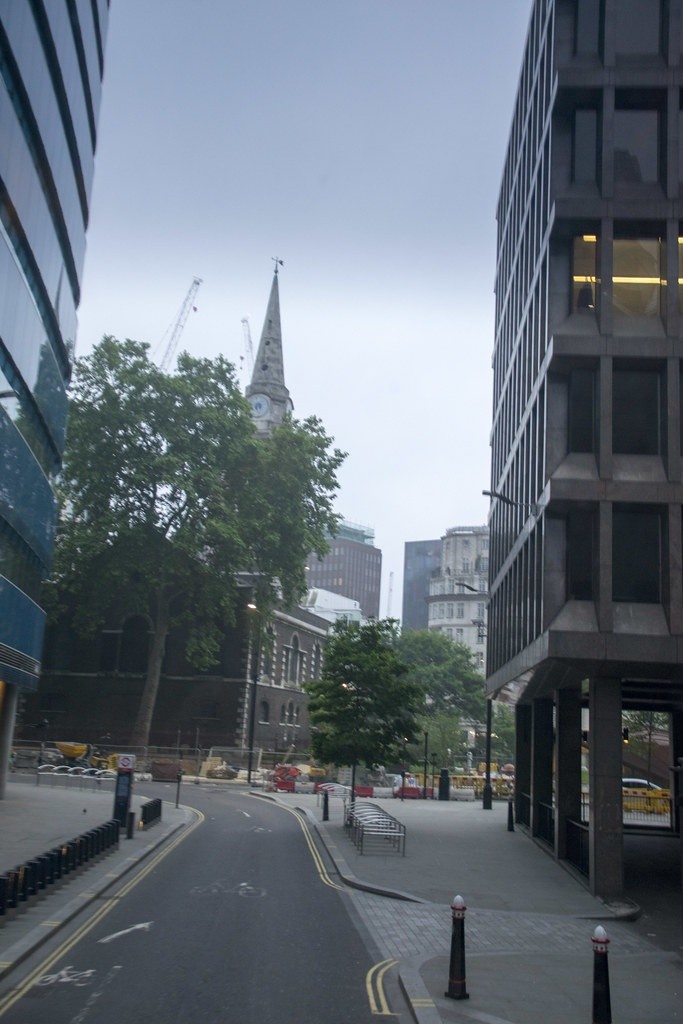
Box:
[622,778,662,807]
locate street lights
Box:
[423,729,430,800]
[400,737,409,802]
[341,681,359,828]
[244,602,259,784]
[453,579,494,813]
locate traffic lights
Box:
[623,728,629,744]
